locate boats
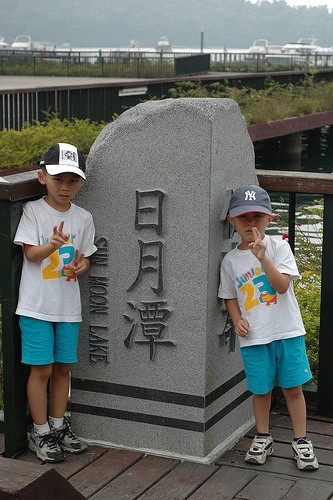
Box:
[156,36,170,52]
[0,33,71,51]
[248,34,333,53]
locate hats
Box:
[229,185,275,220]
[38,142,87,182]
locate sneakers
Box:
[243,435,274,465]
[28,428,65,463]
[290,438,318,470]
[50,416,89,454]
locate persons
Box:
[13,142,97,463]
[217,184,319,470]
